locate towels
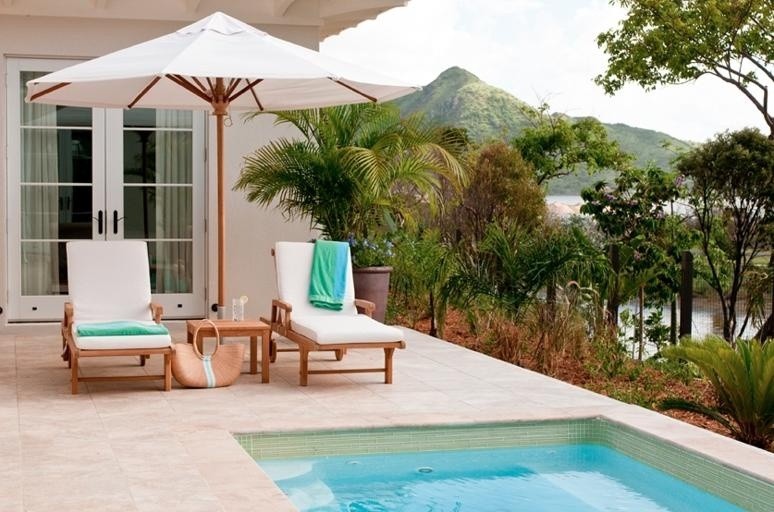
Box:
[75,320,172,338]
[306,237,350,312]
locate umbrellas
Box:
[23,9,422,319]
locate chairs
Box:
[257,238,406,388]
[57,235,176,397]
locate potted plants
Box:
[230,97,476,323]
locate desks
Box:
[183,314,272,385]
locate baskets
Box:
[167,315,247,391]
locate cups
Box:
[232,298,244,321]
